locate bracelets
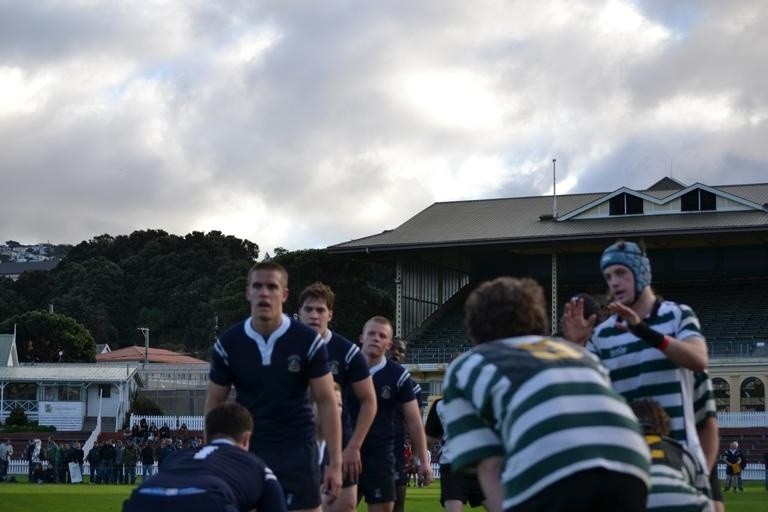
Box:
[660,336,669,350]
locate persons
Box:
[631,398,716,511]
[692,370,722,501]
[559,240,709,487]
[295,278,380,512]
[404,436,431,487]
[726,441,746,491]
[176,424,200,447]
[438,276,649,511]
[27,435,84,483]
[119,402,290,512]
[123,422,170,444]
[87,439,139,485]
[2,438,13,481]
[341,316,435,511]
[203,260,343,512]
[428,398,489,511]
[140,442,155,479]
[155,438,183,467]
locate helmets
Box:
[600,240,653,302]
